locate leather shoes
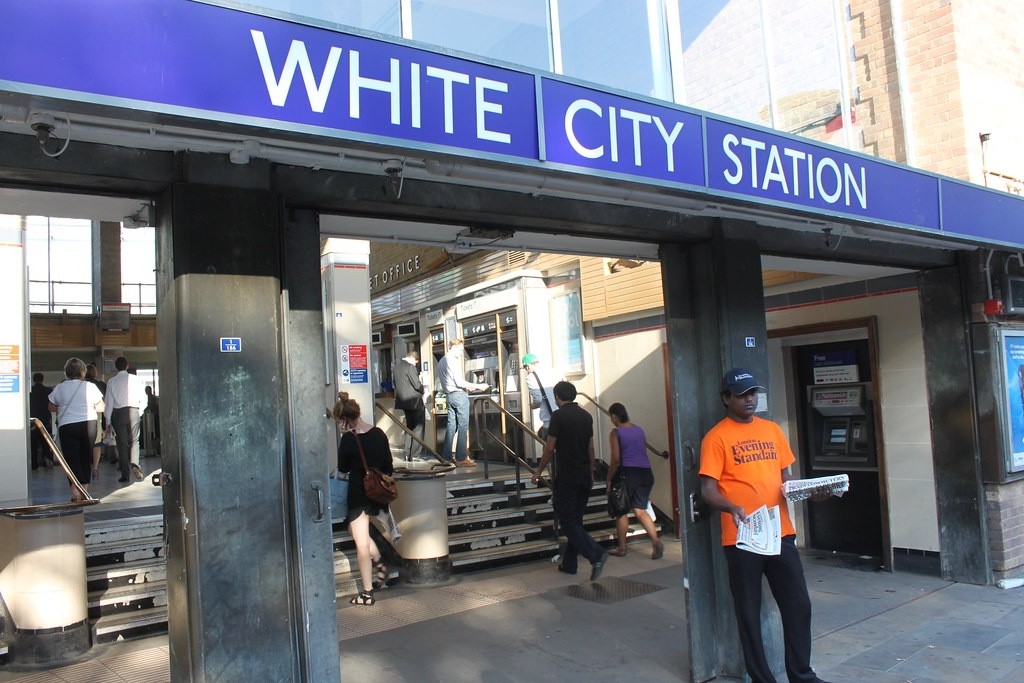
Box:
[118,477,130,482]
[410,454,430,462]
[132,467,145,480]
[404,454,410,461]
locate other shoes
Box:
[608,547,626,557]
[652,542,664,559]
[92,470,99,480]
[109,456,120,464]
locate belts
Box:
[114,407,140,410]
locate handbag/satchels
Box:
[362,467,398,505]
[607,472,632,519]
[329,466,350,519]
[51,427,64,464]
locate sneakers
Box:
[589,551,609,581]
[455,456,478,467]
[558,563,577,574]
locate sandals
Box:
[349,590,375,606]
[373,558,391,588]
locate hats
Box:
[518,354,539,369]
[719,367,766,396]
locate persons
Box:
[521,354,566,506]
[697,368,827,683]
[28,355,160,503]
[531,380,609,582]
[605,403,664,560]
[395,350,433,462]
[333,392,395,606]
[436,339,489,467]
